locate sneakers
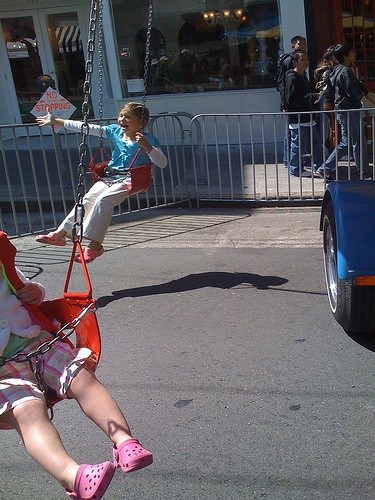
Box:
[314,171,333,181]
[364,177,372,181]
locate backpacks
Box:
[272,53,294,91]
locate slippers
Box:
[75,245,104,263]
[36,232,66,246]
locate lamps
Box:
[202,0,247,29]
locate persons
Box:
[315,46,342,175]
[34,101,168,263]
[0,232,154,500]
[282,52,315,177]
[317,42,375,182]
[135,47,258,92]
[275,35,314,166]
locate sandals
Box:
[113,439,153,473]
[65,461,116,500]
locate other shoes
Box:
[284,166,288,168]
[299,171,311,176]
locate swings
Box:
[0,0,101,430]
[89,0,153,196]
